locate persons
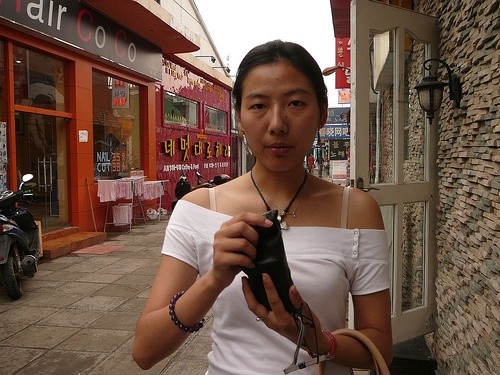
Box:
[307,152,315,176]
[132,40,391,375]
[315,154,324,178]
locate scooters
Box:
[0,172,41,302]
[170,169,231,211]
[194,168,214,187]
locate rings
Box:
[256,311,268,321]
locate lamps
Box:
[196,55,216,63]
[413,58,463,126]
[213,67,231,73]
[322,63,351,76]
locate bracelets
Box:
[168,291,205,333]
[323,331,337,360]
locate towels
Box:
[96,179,117,203]
[142,180,165,201]
[112,202,133,226]
[116,170,144,200]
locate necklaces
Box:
[250,169,308,230]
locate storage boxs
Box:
[214,173,230,185]
